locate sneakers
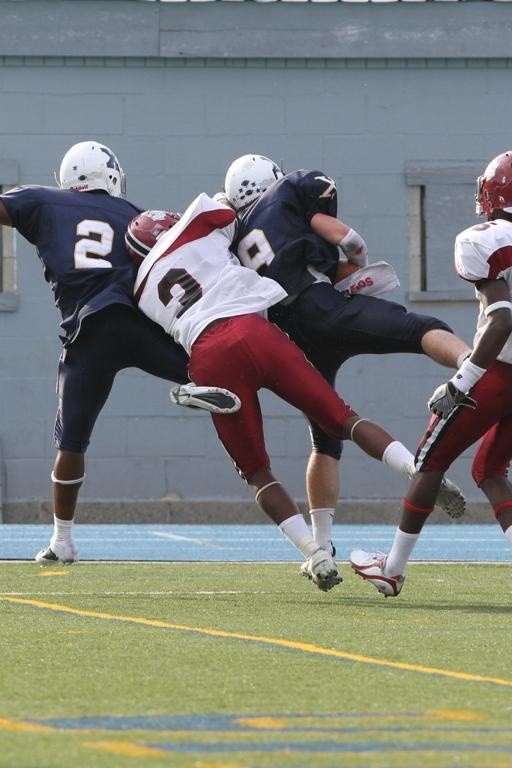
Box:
[404,461,466,519]
[169,386,242,415]
[300,540,343,591]
[33,546,77,568]
[348,550,406,598]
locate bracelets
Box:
[452,359,486,394]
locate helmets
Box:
[58,140,127,200]
[474,151,512,214]
[124,210,180,263]
[224,154,285,219]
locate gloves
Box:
[428,382,477,420]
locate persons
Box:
[349,151,511,596]
[125,190,465,592]
[1,141,243,566]
[225,154,475,578]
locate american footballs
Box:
[335,259,362,283]
